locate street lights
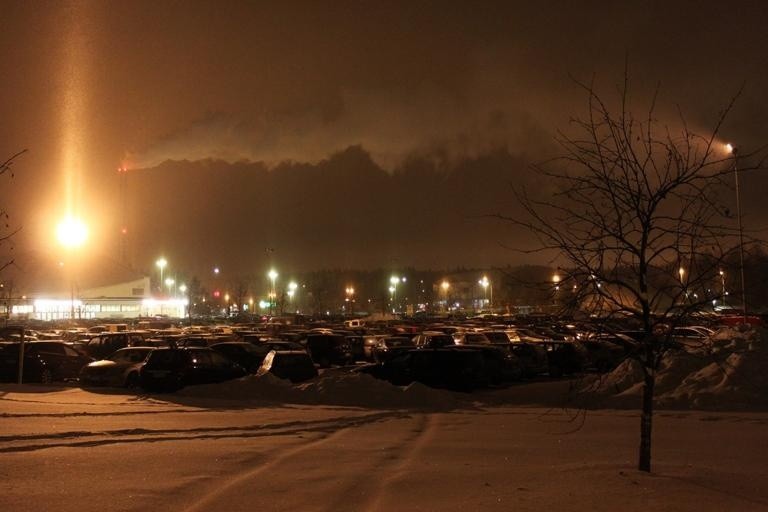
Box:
[724,141,748,328]
